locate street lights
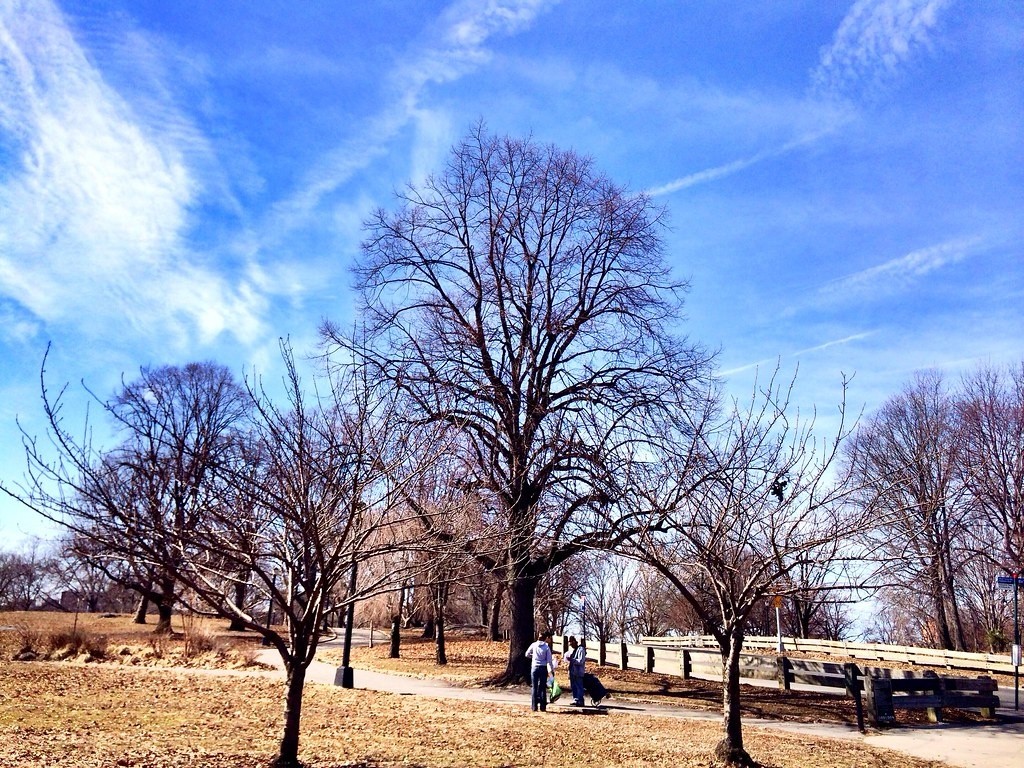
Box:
[333,500,370,689]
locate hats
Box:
[568,636,578,646]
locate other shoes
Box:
[570,702,584,707]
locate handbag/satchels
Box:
[548,679,561,703]
[546,675,554,688]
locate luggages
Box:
[581,671,610,705]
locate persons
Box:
[566,636,585,707]
[525,631,557,712]
